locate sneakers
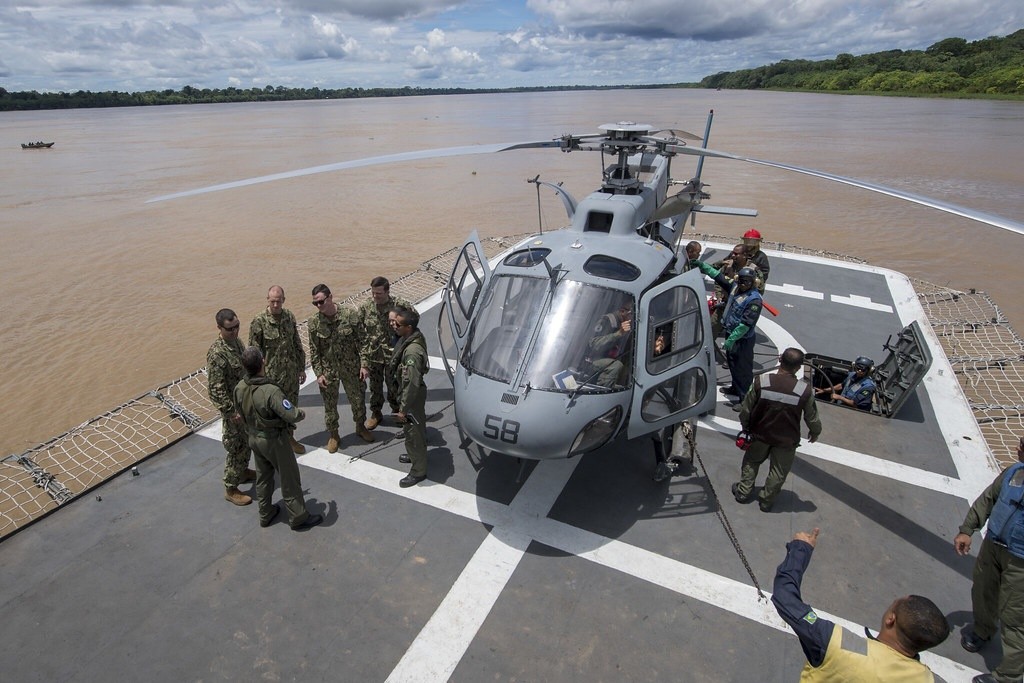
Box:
[733,482,748,503]
[328,410,427,489]
[759,498,773,512]
[247,469,257,481]
[224,490,252,505]
[288,435,306,454]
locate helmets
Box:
[741,229,764,242]
[854,357,871,373]
[737,267,756,285]
[736,430,755,450]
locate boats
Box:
[21,140,54,148]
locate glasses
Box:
[395,321,408,328]
[729,250,742,256]
[855,365,871,374]
[220,322,240,331]
[312,296,329,306]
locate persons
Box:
[390,310,428,488]
[722,229,770,283]
[206,308,257,506]
[583,300,666,387]
[388,304,428,439]
[682,241,701,273]
[308,283,375,453]
[710,243,765,369]
[814,356,876,411]
[357,276,420,429]
[688,258,763,412]
[732,347,822,512]
[248,284,307,453]
[232,346,324,530]
[770,527,950,683]
[954,436,1024,683]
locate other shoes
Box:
[732,402,745,413]
[719,387,737,396]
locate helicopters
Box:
[142,109,1024,483]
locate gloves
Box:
[721,323,749,352]
[689,259,721,279]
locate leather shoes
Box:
[261,503,280,526]
[291,514,323,529]
[722,361,729,369]
[962,629,988,653]
[972,674,1000,683]
